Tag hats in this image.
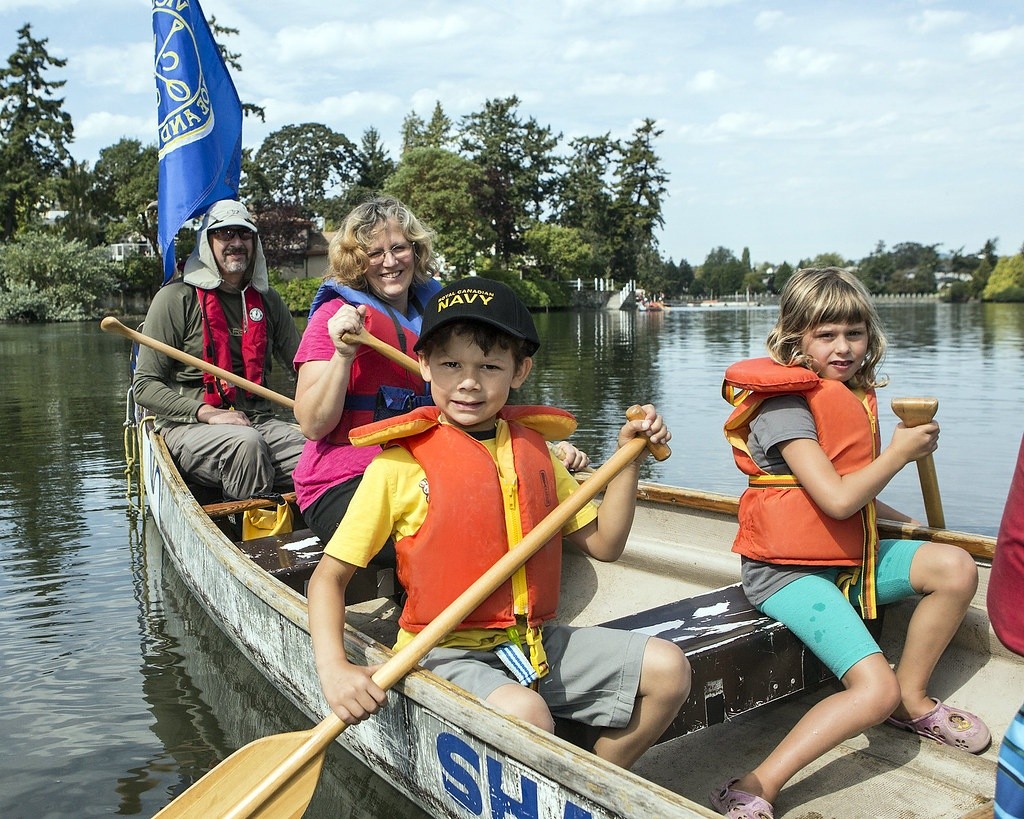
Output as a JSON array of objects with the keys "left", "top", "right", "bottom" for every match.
[
  {"left": 182, "top": 199, "right": 269, "bottom": 292},
  {"left": 412, "top": 276, "right": 540, "bottom": 358}
]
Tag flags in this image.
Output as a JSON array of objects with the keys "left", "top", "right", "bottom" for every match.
[{"left": 153, "top": 0, "right": 243, "bottom": 288}]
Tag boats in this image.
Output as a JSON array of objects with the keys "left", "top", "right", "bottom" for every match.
[{"left": 132, "top": 383, "right": 1024, "bottom": 819}]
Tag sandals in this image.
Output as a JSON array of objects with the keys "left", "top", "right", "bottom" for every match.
[
  {"left": 885, "top": 694, "right": 991, "bottom": 754},
  {"left": 708, "top": 775, "right": 774, "bottom": 819}
]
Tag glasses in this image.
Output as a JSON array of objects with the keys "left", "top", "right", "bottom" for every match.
[
  {"left": 209, "top": 227, "right": 253, "bottom": 241},
  {"left": 367, "top": 242, "right": 416, "bottom": 266}
]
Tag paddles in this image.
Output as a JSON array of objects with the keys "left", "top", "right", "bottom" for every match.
[
  {"left": 147, "top": 399, "right": 674, "bottom": 819},
  {"left": 337, "top": 321, "right": 600, "bottom": 477},
  {"left": 98, "top": 315, "right": 297, "bottom": 411},
  {"left": 886, "top": 394, "right": 953, "bottom": 530}
]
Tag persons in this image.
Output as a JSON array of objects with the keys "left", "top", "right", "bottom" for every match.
[
  {"left": 294, "top": 199, "right": 591, "bottom": 554},
  {"left": 306, "top": 274, "right": 692, "bottom": 772},
  {"left": 131, "top": 199, "right": 303, "bottom": 516},
  {"left": 708, "top": 265, "right": 992, "bottom": 813}
]
